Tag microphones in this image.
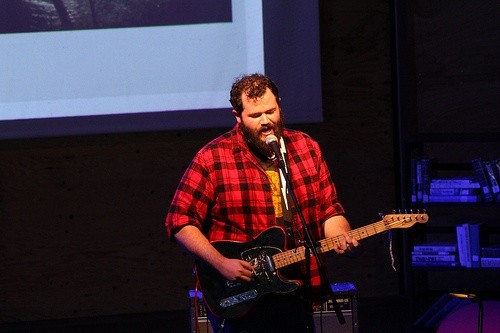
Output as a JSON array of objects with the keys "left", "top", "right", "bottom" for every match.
[{"left": 266, "top": 134, "right": 286, "bottom": 167}]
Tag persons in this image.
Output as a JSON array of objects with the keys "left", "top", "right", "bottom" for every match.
[{"left": 166, "top": 70, "right": 359, "bottom": 333}]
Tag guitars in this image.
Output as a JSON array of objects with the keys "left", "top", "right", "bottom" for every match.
[{"left": 193, "top": 208, "right": 431, "bottom": 321}]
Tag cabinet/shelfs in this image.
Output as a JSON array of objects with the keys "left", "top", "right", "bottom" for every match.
[{"left": 389, "top": 2, "right": 500, "bottom": 331}]
